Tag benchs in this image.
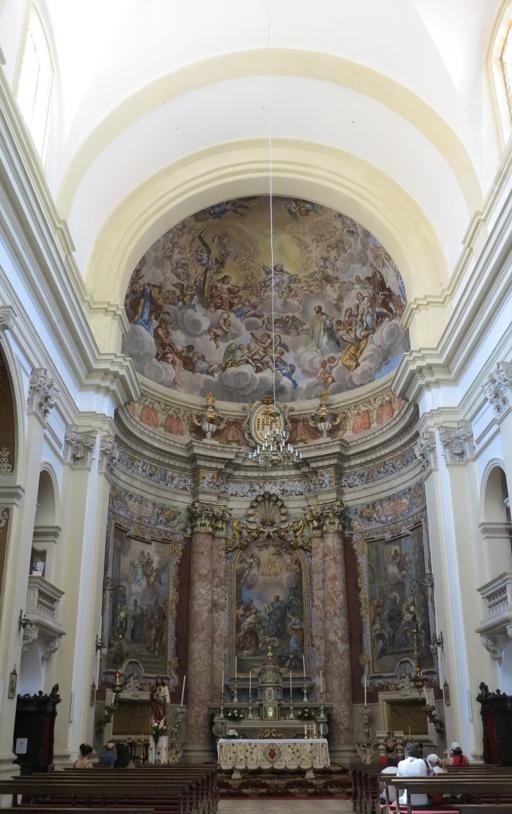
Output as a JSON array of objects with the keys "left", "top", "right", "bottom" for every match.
[
  {"left": 0, "top": 760, "right": 219, "bottom": 813},
  {"left": 351, "top": 761, "right": 512, "bottom": 814}
]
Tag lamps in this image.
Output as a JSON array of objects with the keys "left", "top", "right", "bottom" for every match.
[{"left": 247, "top": 429, "right": 301, "bottom": 470}]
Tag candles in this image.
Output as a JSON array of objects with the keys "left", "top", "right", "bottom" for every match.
[
  {"left": 249, "top": 670, "right": 252, "bottom": 692},
  {"left": 289, "top": 669, "right": 292, "bottom": 695},
  {"left": 319, "top": 670, "right": 323, "bottom": 693},
  {"left": 364, "top": 675, "right": 367, "bottom": 707},
  {"left": 302, "top": 654, "right": 306, "bottom": 682},
  {"left": 234, "top": 656, "right": 238, "bottom": 678},
  {"left": 180, "top": 675, "right": 187, "bottom": 709},
  {"left": 221, "top": 671, "right": 225, "bottom": 692}
]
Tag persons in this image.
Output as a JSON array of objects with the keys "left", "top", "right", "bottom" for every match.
[
  {"left": 114, "top": 745, "right": 135, "bottom": 769},
  {"left": 149, "top": 677, "right": 170, "bottom": 729},
  {"left": 378, "top": 756, "right": 404, "bottom": 805},
  {"left": 98, "top": 742, "right": 116, "bottom": 768},
  {"left": 74, "top": 743, "right": 94, "bottom": 769},
  {"left": 392, "top": 743, "right": 428, "bottom": 806},
  {"left": 444, "top": 743, "right": 469, "bottom": 768},
  {"left": 424, "top": 754, "right": 448, "bottom": 775}
]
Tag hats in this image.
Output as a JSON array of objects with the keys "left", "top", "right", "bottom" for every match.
[{"left": 450, "top": 742, "right": 461, "bottom": 751}]
